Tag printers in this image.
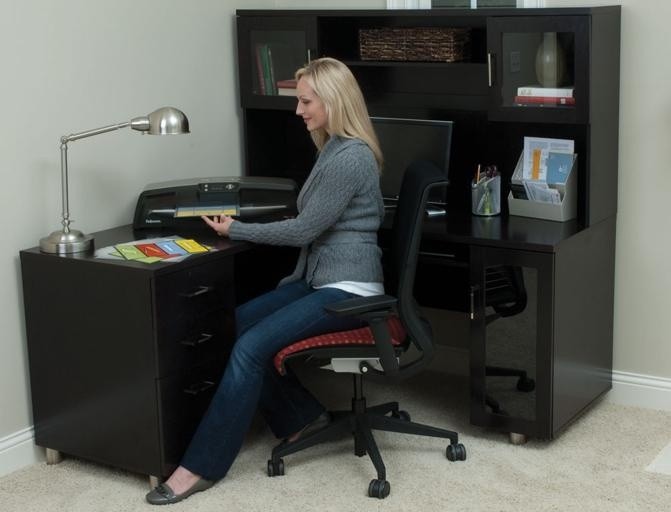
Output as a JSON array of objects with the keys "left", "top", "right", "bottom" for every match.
[{"left": 132, "top": 176, "right": 300, "bottom": 241}]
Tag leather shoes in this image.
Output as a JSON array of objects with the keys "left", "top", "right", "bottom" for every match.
[
  {"left": 272, "top": 412, "right": 328, "bottom": 456},
  {"left": 146, "top": 477, "right": 214, "bottom": 504}
]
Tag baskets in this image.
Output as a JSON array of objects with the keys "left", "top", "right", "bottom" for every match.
[{"left": 359, "top": 27, "right": 463, "bottom": 62}]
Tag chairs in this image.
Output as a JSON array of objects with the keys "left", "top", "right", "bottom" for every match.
[{"left": 265, "top": 158, "right": 467, "bottom": 501}]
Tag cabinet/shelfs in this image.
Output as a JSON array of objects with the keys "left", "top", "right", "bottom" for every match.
[
  {"left": 17, "top": 225, "right": 235, "bottom": 489},
  {"left": 237, "top": 6, "right": 622, "bottom": 225},
  {"left": 463, "top": 226, "right": 616, "bottom": 444}
]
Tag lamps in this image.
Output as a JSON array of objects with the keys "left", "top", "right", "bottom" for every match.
[{"left": 38, "top": 106, "right": 189, "bottom": 256}]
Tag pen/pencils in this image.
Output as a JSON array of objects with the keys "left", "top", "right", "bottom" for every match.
[{"left": 475, "top": 164, "right": 497, "bottom": 215}]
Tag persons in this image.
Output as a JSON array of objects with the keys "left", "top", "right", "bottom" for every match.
[{"left": 145, "top": 57, "right": 389, "bottom": 507}]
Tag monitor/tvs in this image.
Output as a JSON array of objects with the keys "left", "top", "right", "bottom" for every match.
[{"left": 368, "top": 116, "right": 453, "bottom": 217}]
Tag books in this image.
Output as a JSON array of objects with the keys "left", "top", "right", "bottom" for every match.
[
  {"left": 513, "top": 84, "right": 578, "bottom": 111},
  {"left": 250, "top": 39, "right": 303, "bottom": 98}
]
